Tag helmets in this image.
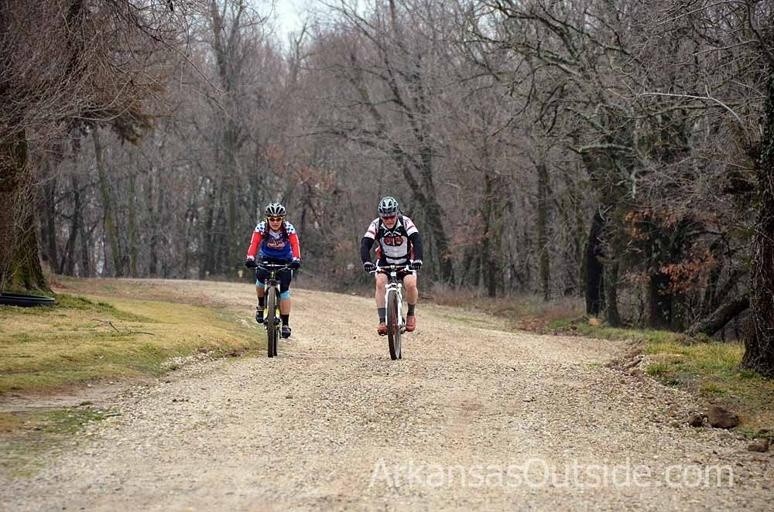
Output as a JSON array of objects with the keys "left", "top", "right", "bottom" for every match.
[
  {"left": 376, "top": 195, "right": 400, "bottom": 218},
  {"left": 263, "top": 202, "right": 287, "bottom": 217}
]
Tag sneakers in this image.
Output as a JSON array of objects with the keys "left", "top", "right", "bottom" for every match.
[
  {"left": 377, "top": 323, "right": 387, "bottom": 335},
  {"left": 406, "top": 312, "right": 416, "bottom": 333},
  {"left": 281, "top": 324, "right": 291, "bottom": 339},
  {"left": 255, "top": 307, "right": 265, "bottom": 323}
]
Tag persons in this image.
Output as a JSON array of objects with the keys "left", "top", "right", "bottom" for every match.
[
  {"left": 244, "top": 201, "right": 301, "bottom": 339},
  {"left": 360, "top": 196, "right": 424, "bottom": 334}
]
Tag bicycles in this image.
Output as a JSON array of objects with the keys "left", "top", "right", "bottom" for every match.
[
  {"left": 369, "top": 262, "right": 420, "bottom": 360},
  {"left": 255, "top": 261, "right": 295, "bottom": 358}
]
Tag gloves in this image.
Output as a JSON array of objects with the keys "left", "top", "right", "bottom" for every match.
[
  {"left": 364, "top": 261, "right": 378, "bottom": 272},
  {"left": 245, "top": 259, "right": 260, "bottom": 271},
  {"left": 409, "top": 258, "right": 422, "bottom": 271},
  {"left": 286, "top": 261, "right": 301, "bottom": 272}
]
